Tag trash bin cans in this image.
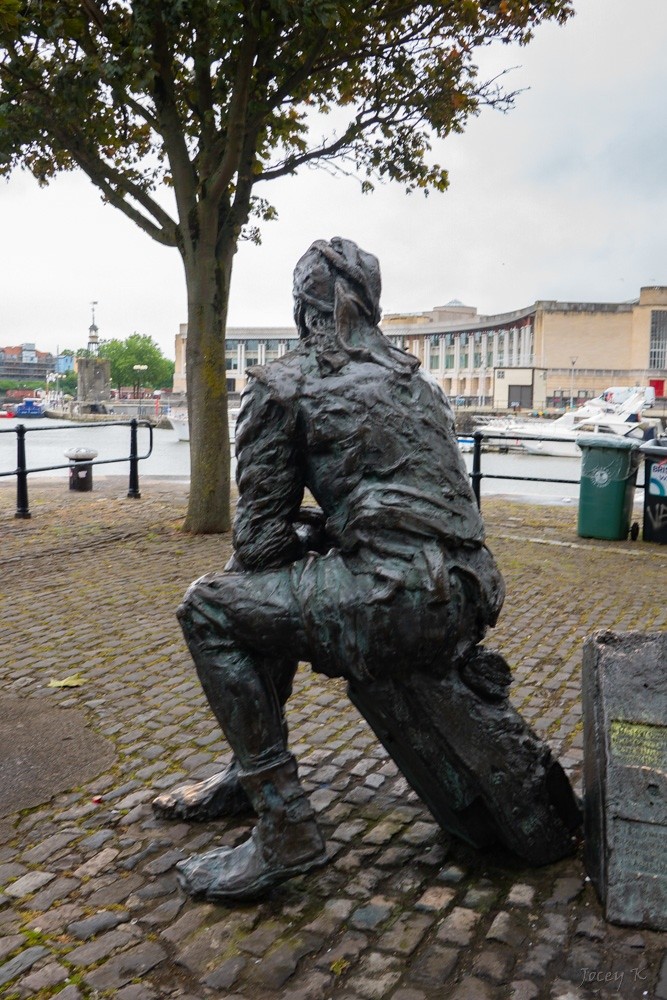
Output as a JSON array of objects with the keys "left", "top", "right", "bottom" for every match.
[
  {"left": 639, "top": 439, "right": 667, "bottom": 543},
  {"left": 576, "top": 437, "right": 641, "bottom": 540},
  {"left": 64, "top": 447, "right": 99, "bottom": 493}
]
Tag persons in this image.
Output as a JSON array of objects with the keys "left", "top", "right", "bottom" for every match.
[{"left": 153, "top": 235, "right": 506, "bottom": 906}]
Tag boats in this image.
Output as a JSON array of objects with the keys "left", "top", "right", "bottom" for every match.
[
  {"left": 17, "top": 398, "right": 46, "bottom": 418},
  {"left": 0, "top": 403, "right": 16, "bottom": 417},
  {"left": 168, "top": 408, "right": 238, "bottom": 444},
  {"left": 475, "top": 387, "right": 667, "bottom": 457},
  {"left": 459, "top": 435, "right": 475, "bottom": 451}
]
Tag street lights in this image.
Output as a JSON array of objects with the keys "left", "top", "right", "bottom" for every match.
[
  {"left": 46, "top": 372, "right": 67, "bottom": 404},
  {"left": 570, "top": 355, "right": 579, "bottom": 407},
  {"left": 133, "top": 365, "right": 148, "bottom": 398}
]
